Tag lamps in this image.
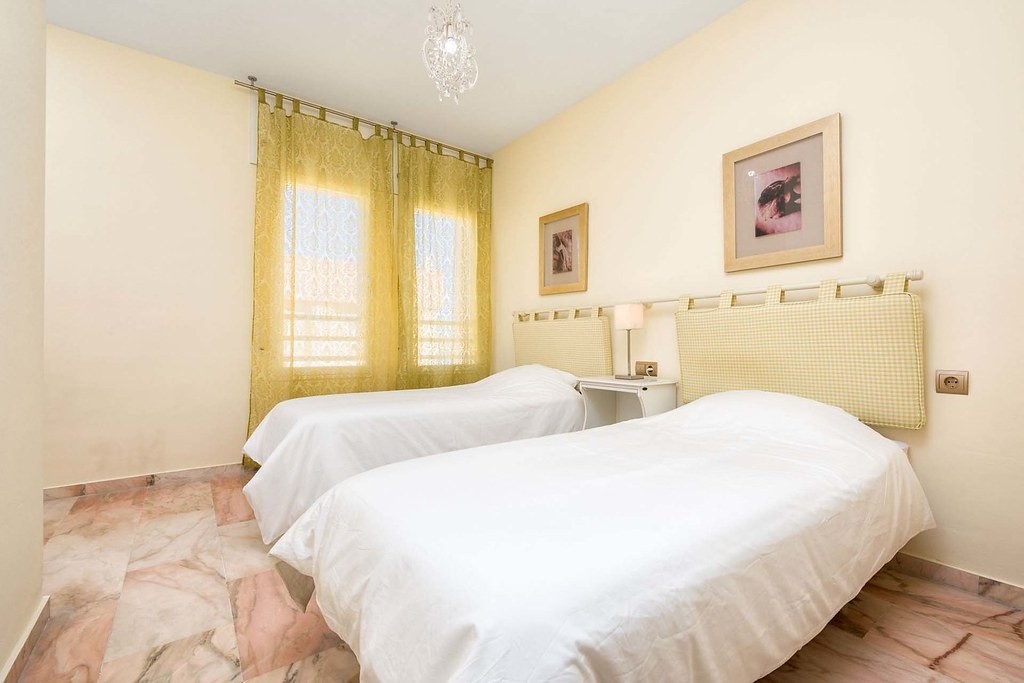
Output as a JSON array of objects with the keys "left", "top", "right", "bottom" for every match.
[
  {"left": 613, "top": 304, "right": 645, "bottom": 380},
  {"left": 421, "top": 0, "right": 479, "bottom": 107}
]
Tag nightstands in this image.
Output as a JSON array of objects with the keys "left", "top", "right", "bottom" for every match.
[{"left": 577, "top": 375, "right": 679, "bottom": 431}]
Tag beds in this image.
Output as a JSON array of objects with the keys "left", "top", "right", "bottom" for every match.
[
  {"left": 239, "top": 306, "right": 614, "bottom": 549},
  {"left": 270, "top": 269, "right": 938, "bottom": 683}
]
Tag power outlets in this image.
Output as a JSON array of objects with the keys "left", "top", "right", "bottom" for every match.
[
  {"left": 635, "top": 361, "right": 657, "bottom": 377},
  {"left": 935, "top": 370, "right": 968, "bottom": 395}
]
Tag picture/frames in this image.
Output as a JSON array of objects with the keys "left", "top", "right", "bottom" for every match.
[
  {"left": 722, "top": 113, "right": 843, "bottom": 273},
  {"left": 539, "top": 203, "right": 589, "bottom": 295}
]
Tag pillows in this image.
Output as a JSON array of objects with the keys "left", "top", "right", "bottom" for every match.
[{"left": 536, "top": 363, "right": 580, "bottom": 388}]
